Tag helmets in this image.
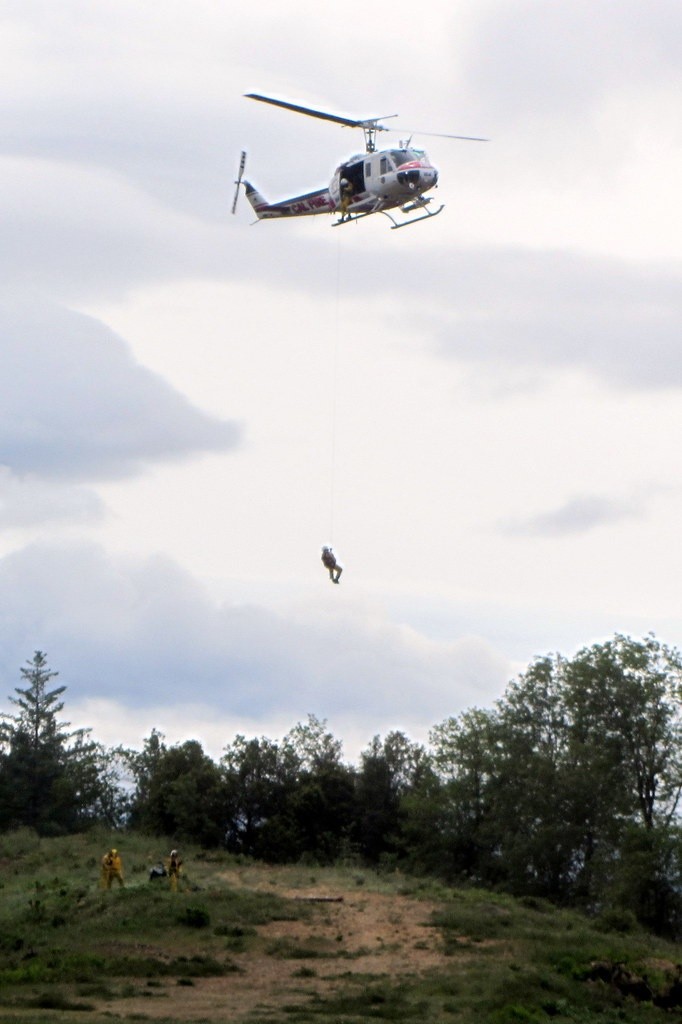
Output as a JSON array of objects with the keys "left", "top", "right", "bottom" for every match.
[
  {"left": 322, "top": 546, "right": 329, "bottom": 551},
  {"left": 112, "top": 849, "right": 117, "bottom": 854},
  {"left": 170, "top": 850, "right": 177, "bottom": 856}
]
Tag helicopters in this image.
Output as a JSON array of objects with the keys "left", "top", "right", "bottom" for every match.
[{"left": 228, "top": 93, "right": 491, "bottom": 230}]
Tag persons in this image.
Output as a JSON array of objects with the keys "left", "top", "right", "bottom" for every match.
[
  {"left": 322, "top": 546, "right": 343, "bottom": 584},
  {"left": 151, "top": 861, "right": 166, "bottom": 879},
  {"left": 337, "top": 178, "right": 353, "bottom": 223},
  {"left": 105, "top": 849, "right": 124, "bottom": 887},
  {"left": 170, "top": 849, "right": 182, "bottom": 891}
]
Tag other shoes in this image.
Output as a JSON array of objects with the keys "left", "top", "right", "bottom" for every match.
[{"left": 332, "top": 579, "right": 339, "bottom": 583}]
